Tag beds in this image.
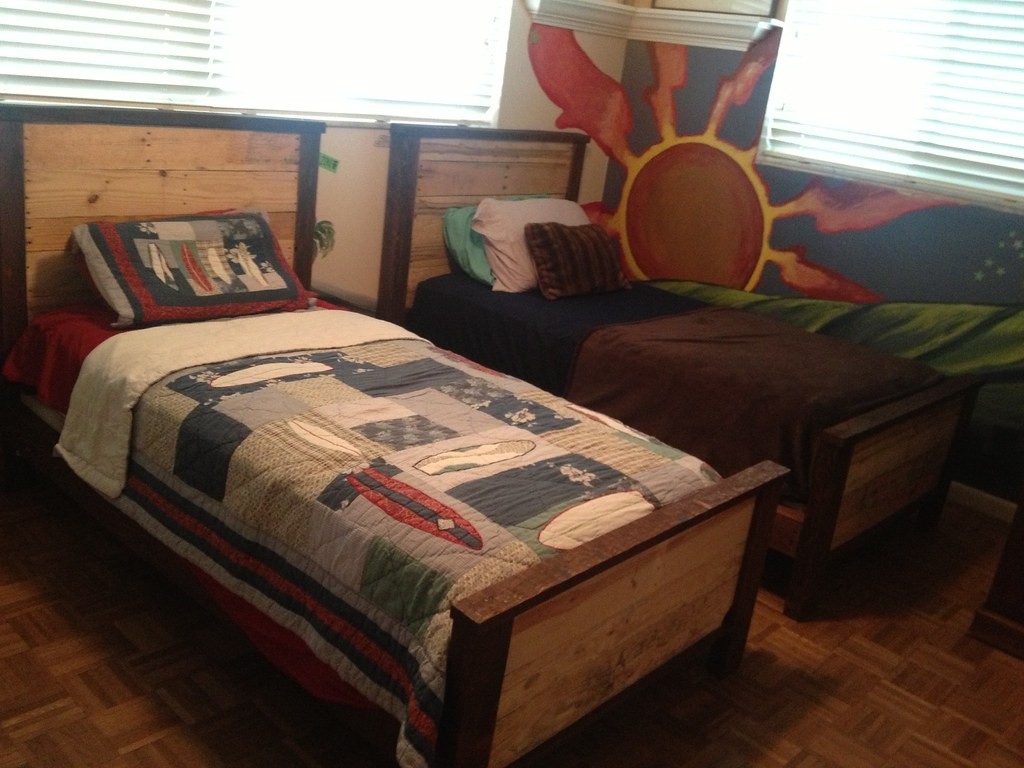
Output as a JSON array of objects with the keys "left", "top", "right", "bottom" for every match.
[
  {"left": 375, "top": 124, "right": 981, "bottom": 620},
  {"left": 0, "top": 104, "right": 793, "bottom": 768}
]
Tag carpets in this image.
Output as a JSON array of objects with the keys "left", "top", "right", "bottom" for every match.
[{"left": 967, "top": 502, "right": 1024, "bottom": 661}]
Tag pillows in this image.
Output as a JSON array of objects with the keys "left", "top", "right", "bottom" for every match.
[
  {"left": 62, "top": 208, "right": 311, "bottom": 328},
  {"left": 440, "top": 195, "right": 550, "bottom": 285},
  {"left": 472, "top": 200, "right": 591, "bottom": 292},
  {"left": 524, "top": 222, "right": 634, "bottom": 301}
]
